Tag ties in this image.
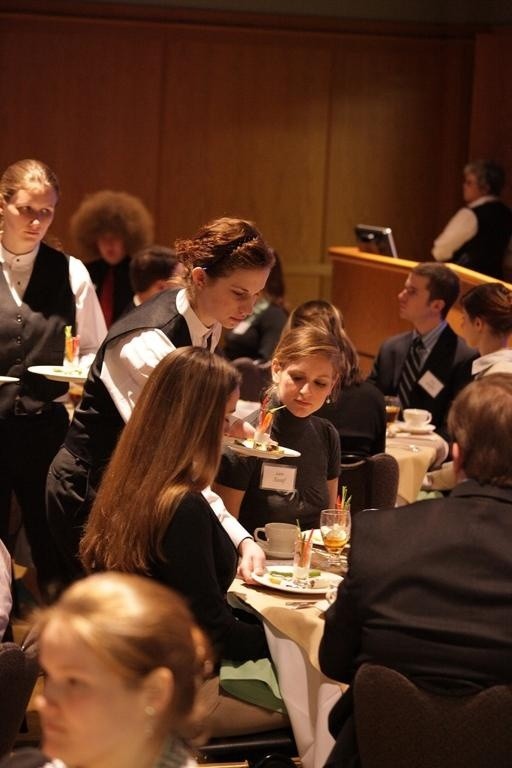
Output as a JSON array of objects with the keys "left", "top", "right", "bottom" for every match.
[{"left": 396, "top": 336, "right": 425, "bottom": 421}]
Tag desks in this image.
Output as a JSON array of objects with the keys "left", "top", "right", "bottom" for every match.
[{"left": 333, "top": 245, "right": 511, "bottom": 381}]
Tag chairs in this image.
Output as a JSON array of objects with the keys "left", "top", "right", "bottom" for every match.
[
  {"left": 352, "top": 662, "right": 512, "bottom": 768},
  {"left": 335, "top": 455, "right": 402, "bottom": 514},
  {"left": 193, "top": 734, "right": 300, "bottom": 766},
  {"left": 0, "top": 639, "right": 40, "bottom": 767},
  {"left": 227, "top": 358, "right": 278, "bottom": 402}
]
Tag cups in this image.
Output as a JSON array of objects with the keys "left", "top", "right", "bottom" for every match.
[
  {"left": 335, "top": 502, "right": 350, "bottom": 526},
  {"left": 403, "top": 409, "right": 432, "bottom": 427},
  {"left": 320, "top": 509, "right": 352, "bottom": 569},
  {"left": 383, "top": 396, "right": 401, "bottom": 439},
  {"left": 293, "top": 539, "right": 313, "bottom": 569},
  {"left": 254, "top": 523, "right": 300, "bottom": 553}
]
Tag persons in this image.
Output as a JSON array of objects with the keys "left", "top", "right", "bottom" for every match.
[
  {"left": 432, "top": 159, "right": 512, "bottom": 279},
  {"left": 211, "top": 329, "right": 342, "bottom": 546},
  {"left": 35, "top": 571, "right": 206, "bottom": 768},
  {"left": 45, "top": 218, "right": 279, "bottom": 584},
  {"left": 79, "top": 347, "right": 290, "bottom": 739},
  {"left": 121, "top": 246, "right": 183, "bottom": 318},
  {"left": 0, "top": 161, "right": 110, "bottom": 602},
  {"left": 321, "top": 375, "right": 512, "bottom": 768},
  {"left": 71, "top": 191, "right": 152, "bottom": 326},
  {"left": 280, "top": 298, "right": 385, "bottom": 458},
  {"left": 363, "top": 262, "right": 481, "bottom": 464},
  {"left": 223, "top": 246, "right": 288, "bottom": 363},
  {"left": 418, "top": 283, "right": 512, "bottom": 491}
]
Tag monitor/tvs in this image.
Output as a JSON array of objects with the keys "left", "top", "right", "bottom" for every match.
[{"left": 355, "top": 223, "right": 398, "bottom": 259}]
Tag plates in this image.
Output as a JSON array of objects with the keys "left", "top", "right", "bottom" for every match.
[
  {"left": 28, "top": 366, "right": 88, "bottom": 383},
  {"left": 251, "top": 565, "right": 344, "bottom": 593},
  {"left": 398, "top": 424, "right": 435, "bottom": 433},
  {"left": 264, "top": 551, "right": 294, "bottom": 558},
  {"left": 224, "top": 438, "right": 301, "bottom": 459},
  {"left": 301, "top": 529, "right": 351, "bottom": 548}
]
